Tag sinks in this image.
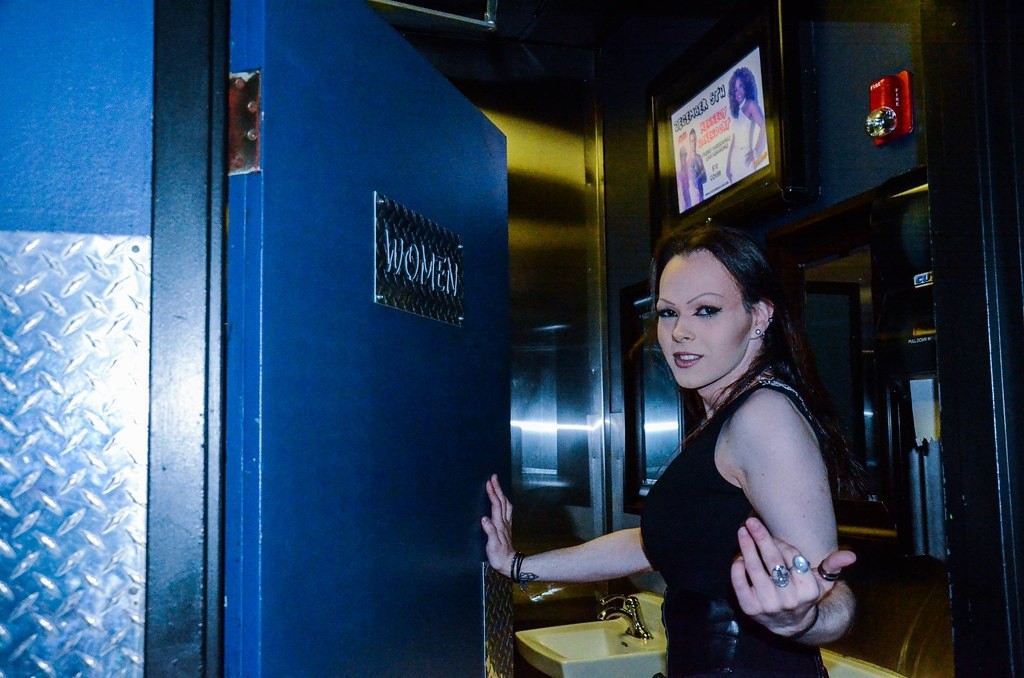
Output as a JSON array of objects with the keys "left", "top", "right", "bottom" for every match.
[{"left": 515, "top": 624, "right": 666, "bottom": 678}]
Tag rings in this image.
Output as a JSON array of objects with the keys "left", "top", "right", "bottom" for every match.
[
  {"left": 770, "top": 564, "right": 791, "bottom": 587},
  {"left": 818, "top": 559, "right": 841, "bottom": 581},
  {"left": 789, "top": 555, "right": 810, "bottom": 573}
]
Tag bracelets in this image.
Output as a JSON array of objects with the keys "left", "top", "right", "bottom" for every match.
[
  {"left": 510, "top": 551, "right": 528, "bottom": 589},
  {"left": 789, "top": 606, "right": 821, "bottom": 640}
]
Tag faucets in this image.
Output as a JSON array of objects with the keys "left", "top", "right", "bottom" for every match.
[{"left": 598, "top": 594, "right": 654, "bottom": 640}]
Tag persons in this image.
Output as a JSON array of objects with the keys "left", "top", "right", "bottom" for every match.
[
  {"left": 482, "top": 226, "right": 856, "bottom": 678},
  {"left": 725, "top": 68, "right": 764, "bottom": 184},
  {"left": 676, "top": 129, "right": 709, "bottom": 212}
]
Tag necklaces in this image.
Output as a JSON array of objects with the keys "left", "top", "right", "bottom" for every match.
[{"left": 701, "top": 367, "right": 770, "bottom": 425}]
